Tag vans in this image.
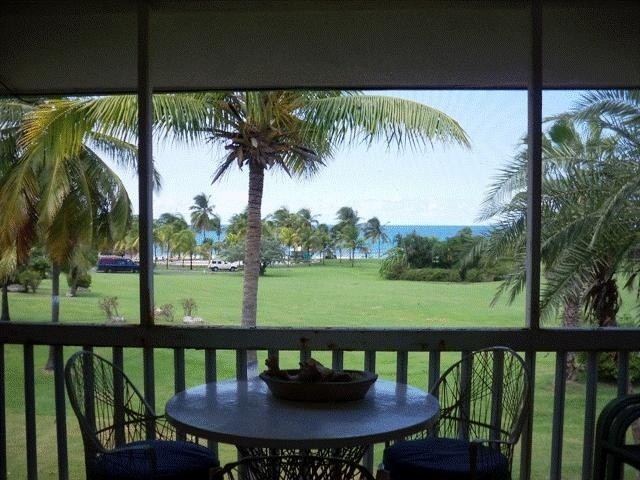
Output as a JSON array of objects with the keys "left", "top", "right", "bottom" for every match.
[{"left": 96, "top": 257, "right": 140, "bottom": 273}]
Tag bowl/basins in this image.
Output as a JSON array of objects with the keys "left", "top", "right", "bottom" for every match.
[{"left": 258, "top": 368, "right": 378, "bottom": 402}]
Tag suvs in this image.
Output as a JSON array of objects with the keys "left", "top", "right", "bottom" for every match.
[{"left": 208, "top": 259, "right": 236, "bottom": 272}]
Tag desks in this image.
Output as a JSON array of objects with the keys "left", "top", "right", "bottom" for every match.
[{"left": 164, "top": 375, "right": 441, "bottom": 464}]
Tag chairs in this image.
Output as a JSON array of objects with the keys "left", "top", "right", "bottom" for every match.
[
  {"left": 375, "top": 346, "right": 533, "bottom": 479},
  {"left": 215, "top": 454, "right": 374, "bottom": 479},
  {"left": 64, "top": 351, "right": 221, "bottom": 479}
]
